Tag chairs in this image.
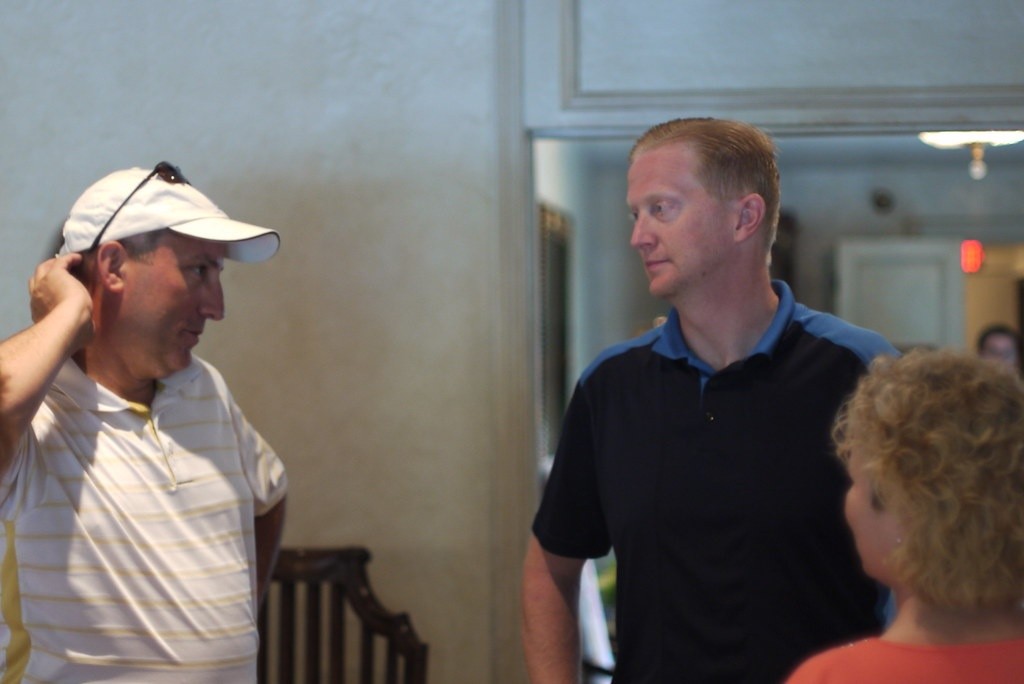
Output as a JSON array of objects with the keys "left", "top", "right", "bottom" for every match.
[{"left": 255, "top": 546, "right": 429, "bottom": 684}]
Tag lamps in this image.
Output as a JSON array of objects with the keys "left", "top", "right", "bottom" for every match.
[{"left": 969, "top": 142, "right": 987, "bottom": 181}]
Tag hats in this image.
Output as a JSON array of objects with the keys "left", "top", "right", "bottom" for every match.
[{"left": 55, "top": 166, "right": 281, "bottom": 265}]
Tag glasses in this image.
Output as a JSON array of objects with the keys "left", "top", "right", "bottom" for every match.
[{"left": 87, "top": 162, "right": 189, "bottom": 253}]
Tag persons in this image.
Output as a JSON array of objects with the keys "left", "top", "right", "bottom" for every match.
[
  {"left": 976, "top": 322, "right": 1016, "bottom": 365},
  {"left": 784, "top": 347, "right": 1024, "bottom": 684},
  {"left": 0, "top": 158, "right": 289, "bottom": 684},
  {"left": 522, "top": 116, "right": 898, "bottom": 684}
]
